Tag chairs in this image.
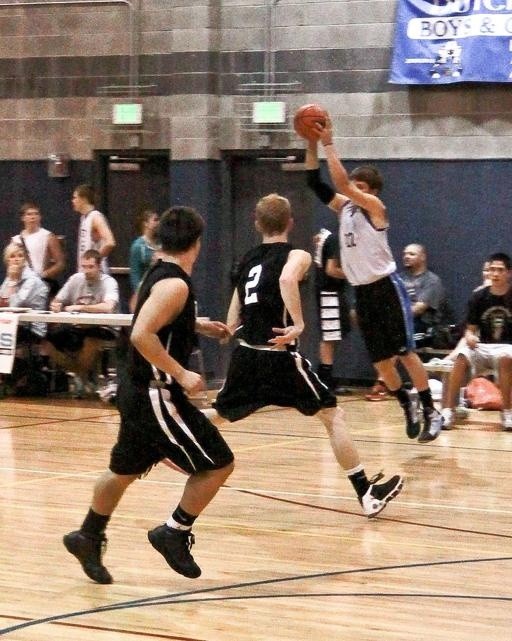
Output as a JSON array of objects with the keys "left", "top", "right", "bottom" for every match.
[
  {"left": 40, "top": 276, "right": 60, "bottom": 308},
  {"left": 189, "top": 300, "right": 207, "bottom": 395}
]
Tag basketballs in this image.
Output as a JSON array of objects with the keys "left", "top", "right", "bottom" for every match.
[{"left": 293, "top": 104, "right": 331, "bottom": 142}]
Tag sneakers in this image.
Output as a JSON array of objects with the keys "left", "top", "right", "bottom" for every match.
[
  {"left": 61, "top": 526, "right": 116, "bottom": 585},
  {"left": 415, "top": 411, "right": 445, "bottom": 447},
  {"left": 401, "top": 398, "right": 422, "bottom": 444},
  {"left": 363, "top": 382, "right": 392, "bottom": 400},
  {"left": 354, "top": 467, "right": 409, "bottom": 524},
  {"left": 496, "top": 406, "right": 512, "bottom": 433},
  {"left": 437, "top": 405, "right": 456, "bottom": 431},
  {"left": 145, "top": 521, "right": 203, "bottom": 580}
]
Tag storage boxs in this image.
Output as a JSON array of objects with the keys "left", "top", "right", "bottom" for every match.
[{"left": 101, "top": 282, "right": 129, "bottom": 377}]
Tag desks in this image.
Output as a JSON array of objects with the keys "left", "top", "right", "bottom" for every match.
[{"left": 0, "top": 310, "right": 133, "bottom": 328}]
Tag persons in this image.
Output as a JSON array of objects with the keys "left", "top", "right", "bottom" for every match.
[
  {"left": 200, "top": 195, "right": 404, "bottom": 519},
  {"left": 365, "top": 243, "right": 444, "bottom": 401},
  {"left": 1, "top": 242, "right": 51, "bottom": 398},
  {"left": 128, "top": 209, "right": 163, "bottom": 293},
  {"left": 7, "top": 202, "right": 66, "bottom": 280},
  {"left": 313, "top": 228, "right": 356, "bottom": 396},
  {"left": 438, "top": 254, "right": 512, "bottom": 430},
  {"left": 50, "top": 249, "right": 121, "bottom": 398},
  {"left": 64, "top": 207, "right": 235, "bottom": 584},
  {"left": 70, "top": 182, "right": 115, "bottom": 274},
  {"left": 306, "top": 114, "right": 447, "bottom": 443}
]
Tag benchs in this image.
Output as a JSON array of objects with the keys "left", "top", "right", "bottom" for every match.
[
  {"left": 415, "top": 348, "right": 456, "bottom": 356},
  {"left": 422, "top": 359, "right": 500, "bottom": 410}
]
